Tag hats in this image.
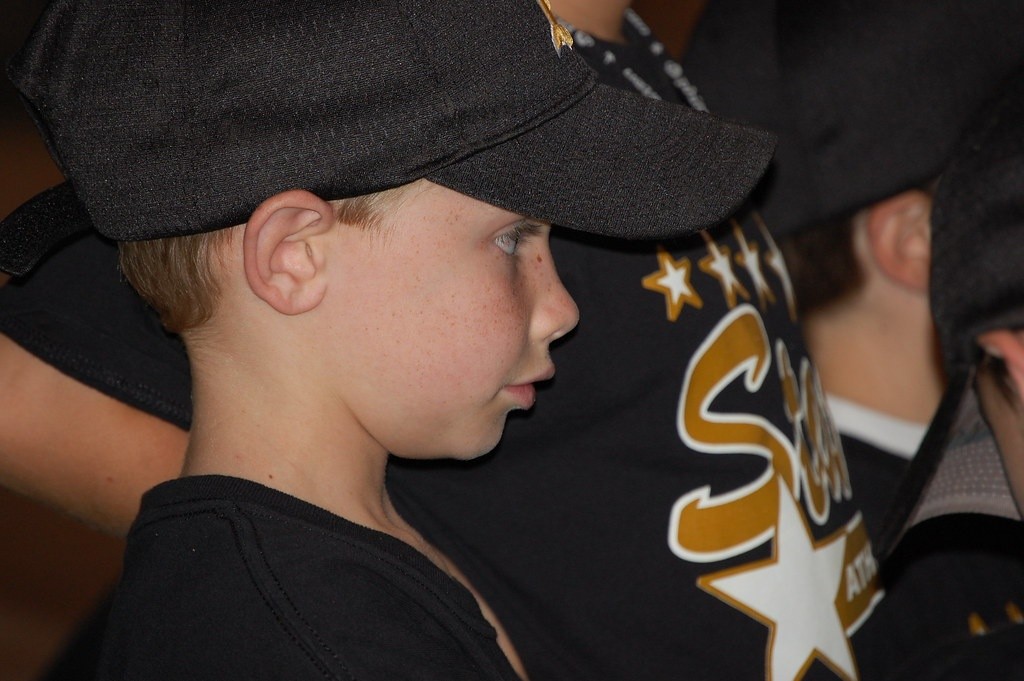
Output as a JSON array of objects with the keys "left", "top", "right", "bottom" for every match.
[
  {"left": 7, "top": 1, "right": 780, "bottom": 239},
  {"left": 931, "top": 88, "right": 1024, "bottom": 388},
  {"left": 678, "top": 0, "right": 1024, "bottom": 234}
]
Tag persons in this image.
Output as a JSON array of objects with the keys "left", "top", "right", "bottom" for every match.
[{"left": 0, "top": 0, "right": 1023, "bottom": 681}]
